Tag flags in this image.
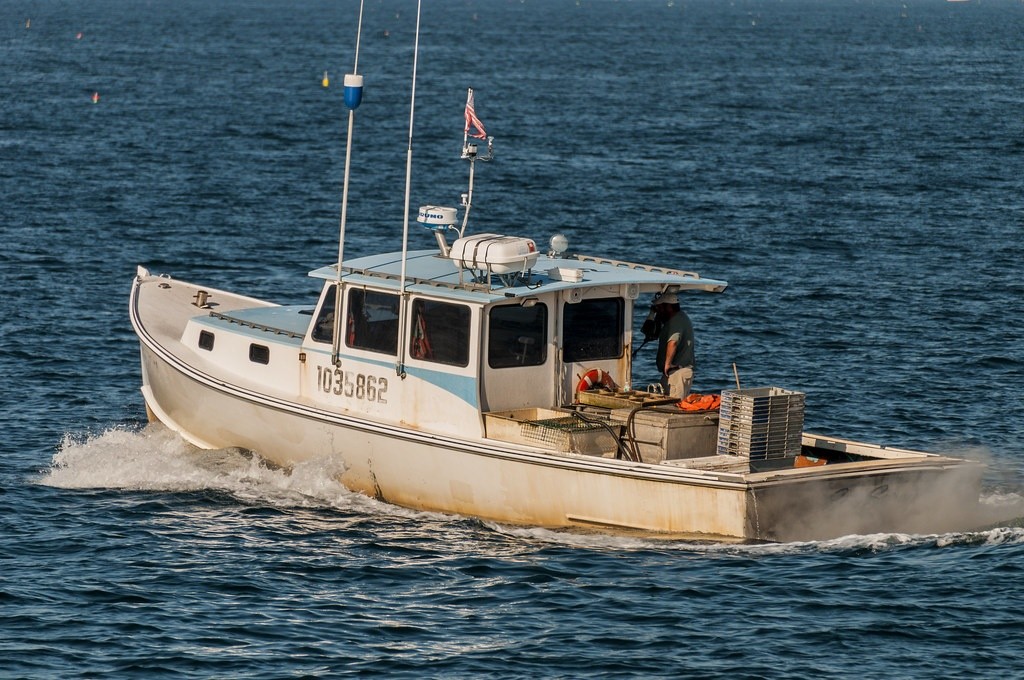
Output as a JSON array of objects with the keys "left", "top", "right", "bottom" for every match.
[{"left": 465, "top": 94, "right": 486, "bottom": 140}]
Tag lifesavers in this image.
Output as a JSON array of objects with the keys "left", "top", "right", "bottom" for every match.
[{"left": 576, "top": 369, "right": 614, "bottom": 397}]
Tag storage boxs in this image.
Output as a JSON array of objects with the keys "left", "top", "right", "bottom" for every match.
[{"left": 483, "top": 386, "right": 805, "bottom": 469}]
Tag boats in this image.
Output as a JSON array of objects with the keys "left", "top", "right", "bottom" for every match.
[{"left": 123, "top": 0, "right": 992, "bottom": 553}]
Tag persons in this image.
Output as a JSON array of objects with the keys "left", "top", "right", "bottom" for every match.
[{"left": 656, "top": 295, "right": 695, "bottom": 401}]
